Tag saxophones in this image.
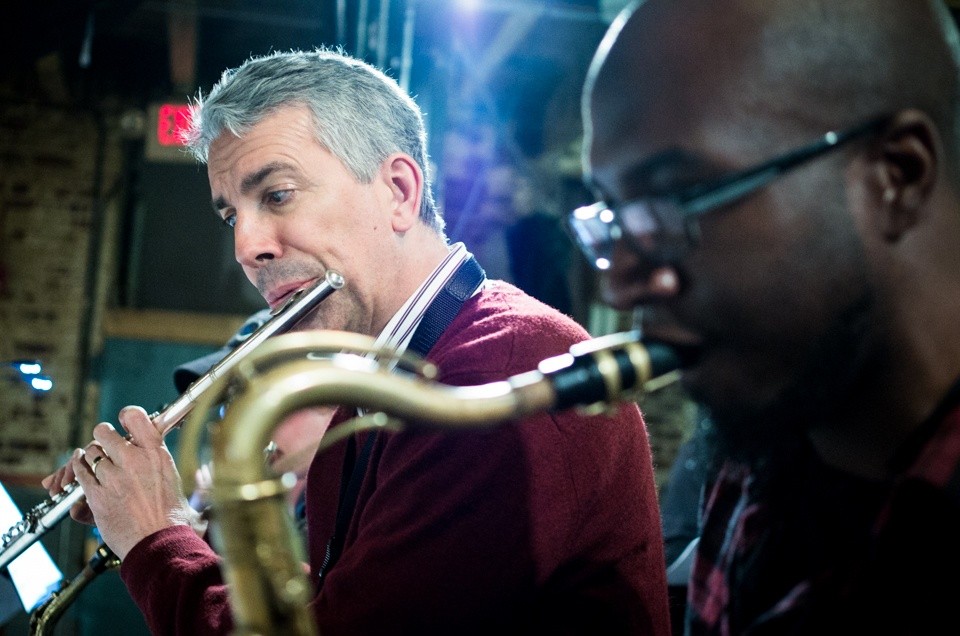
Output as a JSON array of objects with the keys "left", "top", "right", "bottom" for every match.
[{"left": 180, "top": 326, "right": 686, "bottom": 636}]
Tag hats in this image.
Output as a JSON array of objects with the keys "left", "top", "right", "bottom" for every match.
[{"left": 174, "top": 308, "right": 276, "bottom": 395}]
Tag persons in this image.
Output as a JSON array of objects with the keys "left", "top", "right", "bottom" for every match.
[{"left": 42, "top": 0, "right": 960, "bottom": 636}]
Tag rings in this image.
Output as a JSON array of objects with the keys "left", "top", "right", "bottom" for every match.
[{"left": 90, "top": 455, "right": 108, "bottom": 475}]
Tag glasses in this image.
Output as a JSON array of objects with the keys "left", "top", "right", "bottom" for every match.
[{"left": 559, "top": 102, "right": 945, "bottom": 275}]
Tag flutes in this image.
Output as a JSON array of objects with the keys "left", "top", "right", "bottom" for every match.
[{"left": 0, "top": 268, "right": 347, "bottom": 572}]
